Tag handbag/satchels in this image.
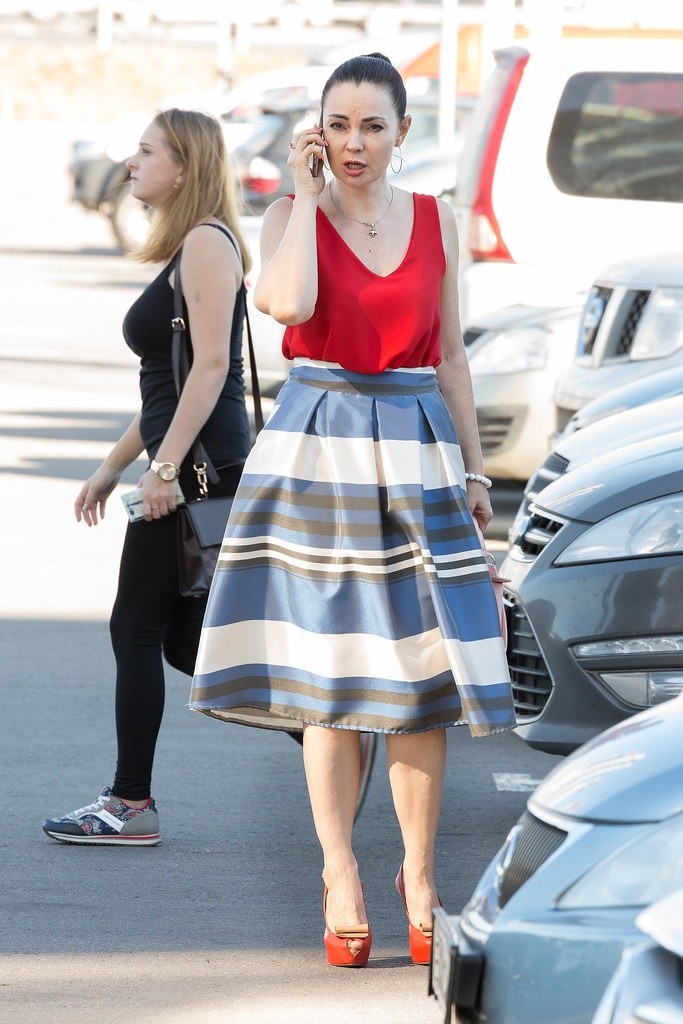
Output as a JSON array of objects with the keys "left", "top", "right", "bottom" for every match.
[
  {"left": 175, "top": 495, "right": 235, "bottom": 598},
  {"left": 471, "top": 512, "right": 508, "bottom": 653}
]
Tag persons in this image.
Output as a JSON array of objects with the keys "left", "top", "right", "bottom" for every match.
[
  {"left": 44, "top": 109, "right": 379, "bottom": 846},
  {"left": 188, "top": 52, "right": 518, "bottom": 969}
]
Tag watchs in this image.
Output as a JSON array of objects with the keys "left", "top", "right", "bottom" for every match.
[{"left": 151, "top": 460, "right": 180, "bottom": 481}]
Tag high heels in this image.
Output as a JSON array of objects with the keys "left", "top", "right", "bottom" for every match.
[
  {"left": 323, "top": 880, "right": 372, "bottom": 967},
  {"left": 395, "top": 864, "right": 444, "bottom": 965}
]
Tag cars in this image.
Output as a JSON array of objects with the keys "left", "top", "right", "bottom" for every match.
[
  {"left": 429, "top": 685, "right": 683, "bottom": 1024},
  {"left": 459, "top": 36, "right": 683, "bottom": 493},
  {"left": 99, "top": 77, "right": 492, "bottom": 263},
  {"left": 490, "top": 250, "right": 683, "bottom": 758}
]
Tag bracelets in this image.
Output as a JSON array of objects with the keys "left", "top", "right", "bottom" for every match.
[{"left": 465, "top": 472, "right": 492, "bottom": 488}]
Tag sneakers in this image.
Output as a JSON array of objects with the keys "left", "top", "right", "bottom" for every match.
[
  {"left": 42, "top": 786, "right": 162, "bottom": 846},
  {"left": 353, "top": 732, "right": 376, "bottom": 826}
]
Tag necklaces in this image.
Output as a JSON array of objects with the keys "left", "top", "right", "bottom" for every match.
[{"left": 328, "top": 179, "right": 394, "bottom": 237}]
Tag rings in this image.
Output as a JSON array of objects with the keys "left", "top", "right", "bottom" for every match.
[{"left": 289, "top": 143, "right": 295, "bottom": 149}]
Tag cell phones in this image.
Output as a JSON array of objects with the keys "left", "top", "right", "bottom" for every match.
[
  {"left": 122, "top": 478, "right": 185, "bottom": 523},
  {"left": 312, "top": 113, "right": 325, "bottom": 178}
]
